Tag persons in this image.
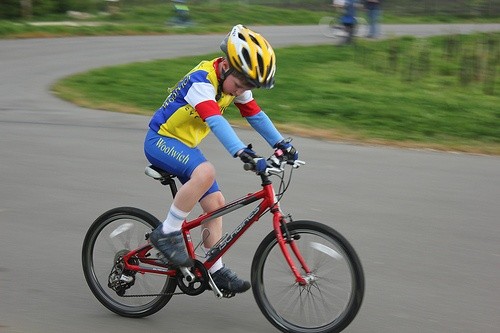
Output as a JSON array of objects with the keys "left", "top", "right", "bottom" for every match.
[
  {"left": 333, "top": 0, "right": 380, "bottom": 47},
  {"left": 144, "top": 22, "right": 301, "bottom": 294},
  {"left": 172, "top": 0, "right": 190, "bottom": 21}
]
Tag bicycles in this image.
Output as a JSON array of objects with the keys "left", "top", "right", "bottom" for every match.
[
  {"left": 82, "top": 138, "right": 365, "bottom": 333},
  {"left": 320, "top": 8, "right": 371, "bottom": 39}
]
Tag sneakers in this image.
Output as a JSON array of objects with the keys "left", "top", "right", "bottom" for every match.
[
  {"left": 147, "top": 223, "right": 194, "bottom": 267},
  {"left": 206, "top": 263, "right": 251, "bottom": 294}
]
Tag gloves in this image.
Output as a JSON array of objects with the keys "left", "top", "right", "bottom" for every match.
[
  {"left": 239, "top": 143, "right": 267, "bottom": 174},
  {"left": 277, "top": 140, "right": 298, "bottom": 161}
]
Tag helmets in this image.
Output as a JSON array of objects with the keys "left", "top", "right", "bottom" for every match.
[{"left": 220, "top": 24, "right": 276, "bottom": 89}]
